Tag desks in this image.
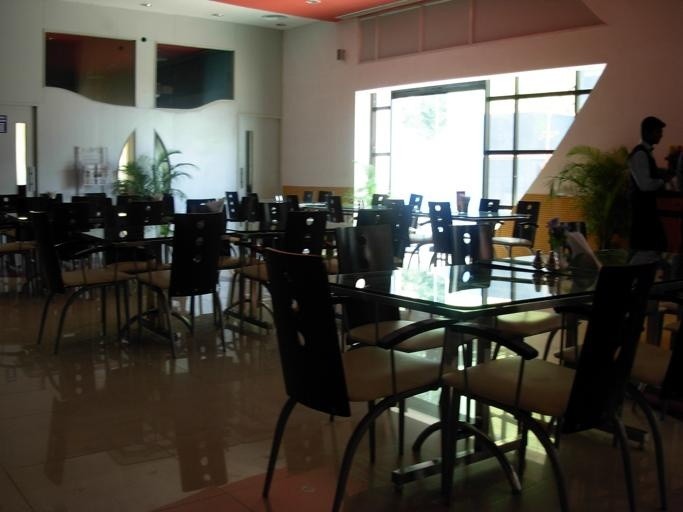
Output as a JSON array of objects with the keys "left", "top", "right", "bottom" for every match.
[{"left": 327, "top": 249, "right": 683, "bottom": 492}]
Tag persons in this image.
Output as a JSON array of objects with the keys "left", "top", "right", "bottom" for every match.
[{"left": 626, "top": 116, "right": 676, "bottom": 251}]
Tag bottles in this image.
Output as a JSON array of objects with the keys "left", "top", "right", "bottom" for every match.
[
  {"left": 531, "top": 249, "right": 543, "bottom": 272},
  {"left": 543, "top": 247, "right": 561, "bottom": 272}
]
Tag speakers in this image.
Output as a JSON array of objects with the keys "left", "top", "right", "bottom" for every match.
[{"left": 337, "top": 49, "right": 345, "bottom": 61}]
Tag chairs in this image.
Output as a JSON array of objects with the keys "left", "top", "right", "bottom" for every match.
[
  {"left": 448, "top": 261, "right": 668, "bottom": 512},
  {"left": 449, "top": 223, "right": 566, "bottom": 445},
  {"left": 331, "top": 222, "right": 471, "bottom": 457},
  {"left": 261, "top": 245, "right": 443, "bottom": 512},
  {"left": 270, "top": 187, "right": 541, "bottom": 254}
]
